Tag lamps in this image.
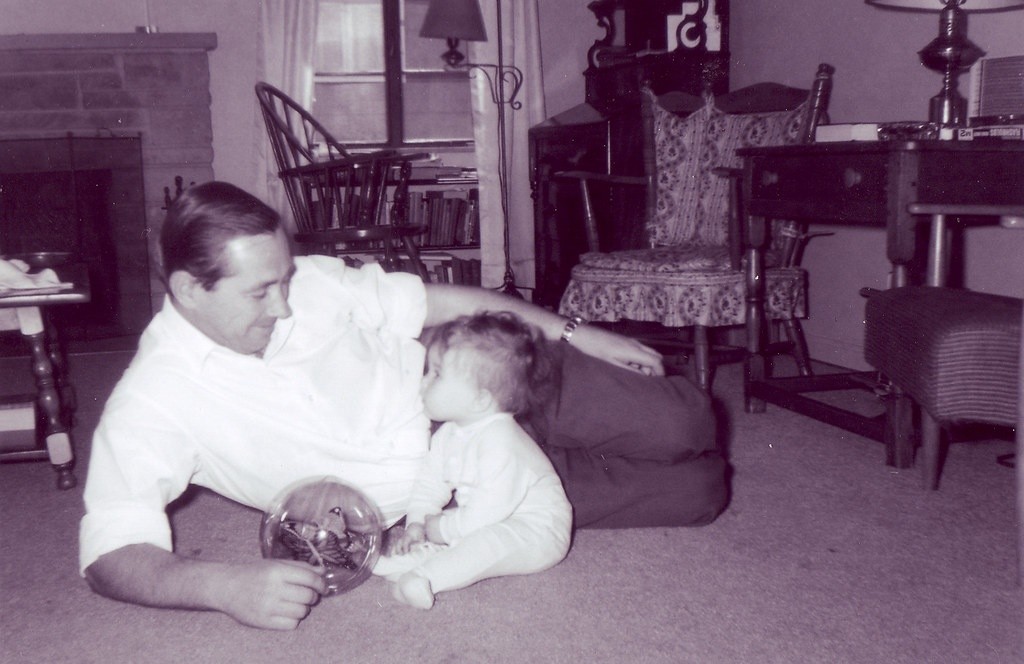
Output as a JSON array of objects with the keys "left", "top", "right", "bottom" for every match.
[
  {"left": 861, "top": 0, "right": 1024, "bottom": 128},
  {"left": 418, "top": 0, "right": 525, "bottom": 300}
]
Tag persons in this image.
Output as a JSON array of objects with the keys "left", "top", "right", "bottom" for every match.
[
  {"left": 372, "top": 313, "right": 575, "bottom": 609},
  {"left": 77, "top": 181, "right": 732, "bottom": 632}
]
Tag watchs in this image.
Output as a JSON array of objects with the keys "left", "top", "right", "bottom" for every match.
[{"left": 560, "top": 314, "right": 588, "bottom": 343}]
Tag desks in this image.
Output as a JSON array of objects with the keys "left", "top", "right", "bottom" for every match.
[
  {"left": 732, "top": 135, "right": 1024, "bottom": 469},
  {"left": 0, "top": 249, "right": 91, "bottom": 491}
]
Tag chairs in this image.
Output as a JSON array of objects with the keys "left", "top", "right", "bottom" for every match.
[
  {"left": 865, "top": 202, "right": 1024, "bottom": 493},
  {"left": 254, "top": 80, "right": 441, "bottom": 283},
  {"left": 551, "top": 57, "right": 836, "bottom": 400}
]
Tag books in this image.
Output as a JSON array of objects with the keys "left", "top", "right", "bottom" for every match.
[
  {"left": 308, "top": 152, "right": 481, "bottom": 291},
  {"left": 815, "top": 121, "right": 955, "bottom": 142},
  {"left": 958, "top": 124, "right": 1024, "bottom": 141}
]
